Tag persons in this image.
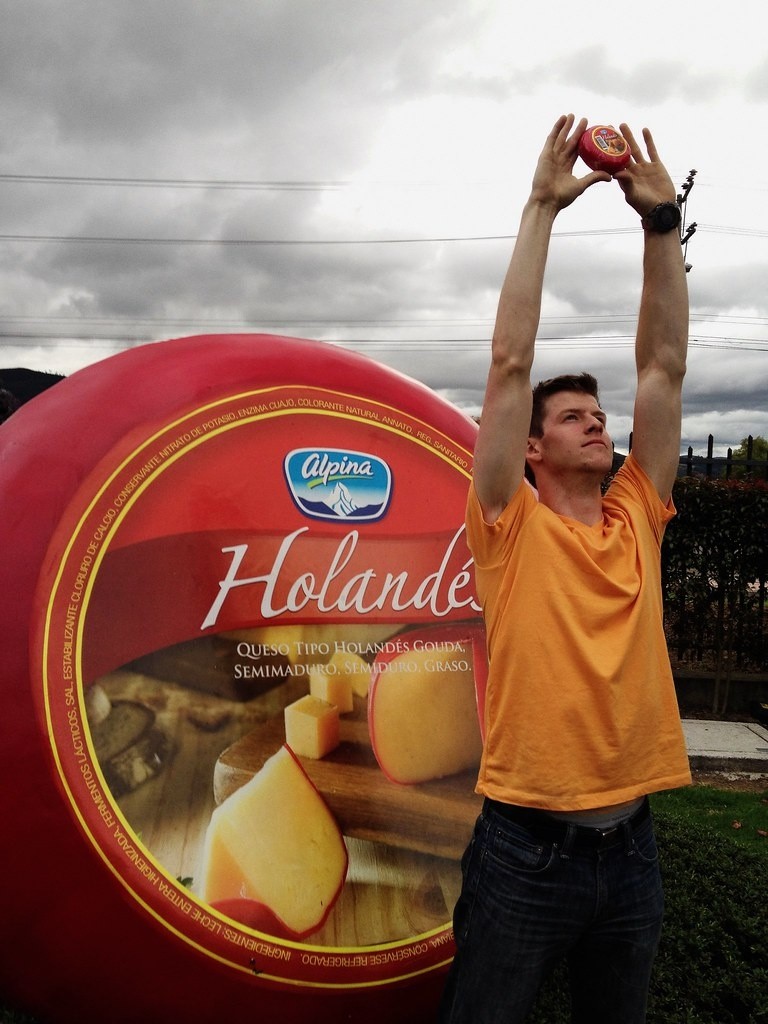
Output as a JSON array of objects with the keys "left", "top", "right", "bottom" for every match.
[{"left": 442, "top": 113, "right": 690, "bottom": 1024}]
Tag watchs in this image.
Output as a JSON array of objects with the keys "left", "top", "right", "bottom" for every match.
[{"left": 640, "top": 203, "right": 682, "bottom": 235}]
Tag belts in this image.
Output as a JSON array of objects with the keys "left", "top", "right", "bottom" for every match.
[{"left": 490, "top": 795, "right": 651, "bottom": 854}]
